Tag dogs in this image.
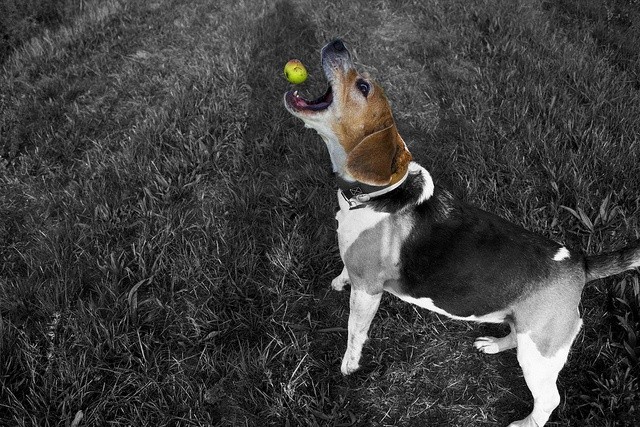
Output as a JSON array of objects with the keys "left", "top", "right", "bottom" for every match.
[{"left": 281, "top": 38, "right": 640, "bottom": 427}]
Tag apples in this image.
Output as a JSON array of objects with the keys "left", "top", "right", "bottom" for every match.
[{"left": 284, "top": 59, "right": 308, "bottom": 86}]
